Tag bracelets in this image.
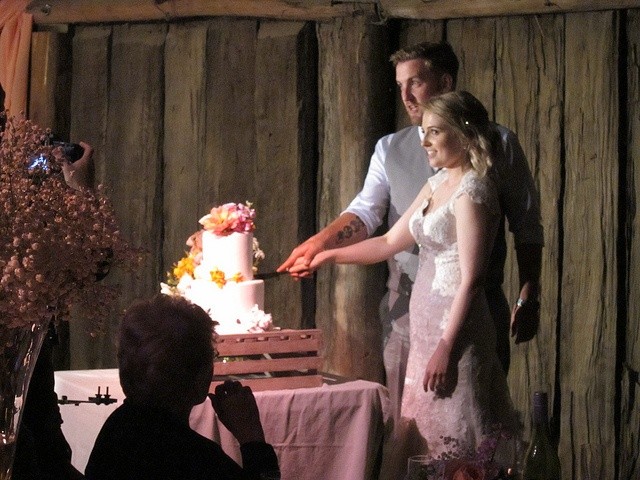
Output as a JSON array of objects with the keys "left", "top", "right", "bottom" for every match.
[{"left": 515, "top": 297, "right": 540, "bottom": 313}]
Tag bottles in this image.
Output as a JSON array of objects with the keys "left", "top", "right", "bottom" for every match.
[{"left": 521, "top": 391, "right": 563, "bottom": 480}]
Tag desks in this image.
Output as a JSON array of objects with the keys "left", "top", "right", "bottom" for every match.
[{"left": 13, "top": 368, "right": 389, "bottom": 477}]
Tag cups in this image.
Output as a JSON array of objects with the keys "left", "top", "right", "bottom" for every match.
[
  {"left": 579, "top": 441, "right": 608, "bottom": 480},
  {"left": 407, "top": 453, "right": 446, "bottom": 479}
]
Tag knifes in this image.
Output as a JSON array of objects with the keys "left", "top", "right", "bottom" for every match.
[{"left": 254, "top": 271, "right": 290, "bottom": 280}]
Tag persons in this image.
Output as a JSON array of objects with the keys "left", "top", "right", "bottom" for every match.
[
  {"left": 284, "top": 89, "right": 521, "bottom": 478},
  {"left": 275, "top": 38, "right": 545, "bottom": 478},
  {"left": 1, "top": 81, "right": 114, "bottom": 480},
  {"left": 83, "top": 292, "right": 283, "bottom": 479}
]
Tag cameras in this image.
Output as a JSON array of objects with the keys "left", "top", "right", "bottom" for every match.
[{"left": 29, "top": 134, "right": 95, "bottom": 176}]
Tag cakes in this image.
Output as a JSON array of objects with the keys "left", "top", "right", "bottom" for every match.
[{"left": 159, "top": 199, "right": 275, "bottom": 336}]
